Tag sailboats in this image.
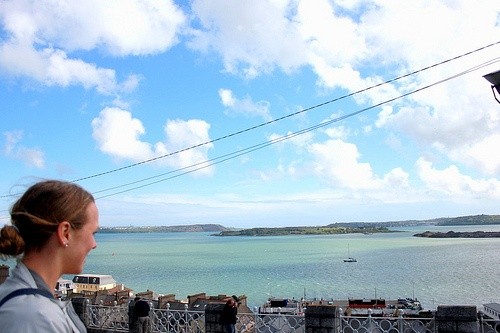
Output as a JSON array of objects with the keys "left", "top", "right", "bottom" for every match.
[{"left": 344, "top": 244, "right": 357, "bottom": 262}]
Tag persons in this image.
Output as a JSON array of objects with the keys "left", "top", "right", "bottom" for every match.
[
  {"left": 222, "top": 299, "right": 237, "bottom": 333},
  {"left": 134, "top": 297, "right": 150, "bottom": 333},
  {"left": 0, "top": 180, "right": 100, "bottom": 333}
]
